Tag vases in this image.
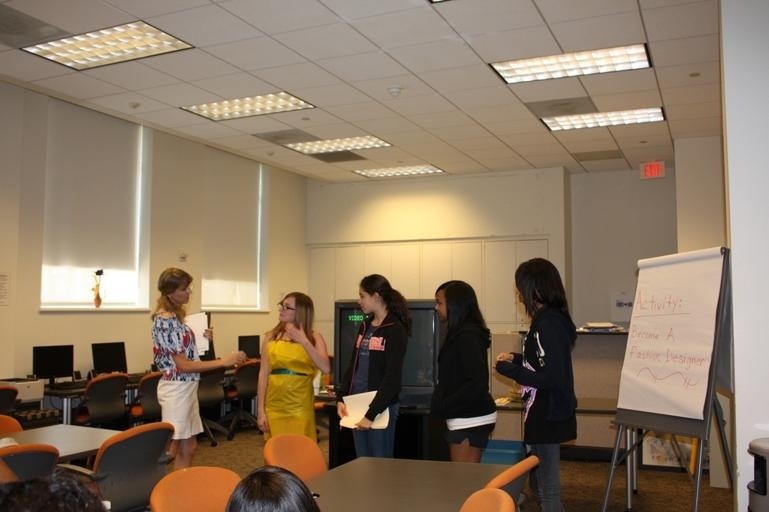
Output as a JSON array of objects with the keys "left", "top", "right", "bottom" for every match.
[{"left": 94, "top": 296, "right": 102, "bottom": 308}]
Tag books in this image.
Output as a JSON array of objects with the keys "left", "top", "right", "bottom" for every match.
[{"left": 576, "top": 322, "right": 624, "bottom": 333}]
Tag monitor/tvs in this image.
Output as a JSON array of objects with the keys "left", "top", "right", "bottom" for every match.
[
  {"left": 30, "top": 343, "right": 74, "bottom": 388},
  {"left": 237, "top": 333, "right": 261, "bottom": 359},
  {"left": 334, "top": 299, "right": 446, "bottom": 410},
  {"left": 92, "top": 341, "right": 129, "bottom": 375}
]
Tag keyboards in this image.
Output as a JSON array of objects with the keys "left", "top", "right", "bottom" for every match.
[
  {"left": 43, "top": 381, "right": 86, "bottom": 389},
  {"left": 128, "top": 377, "right": 142, "bottom": 384}
]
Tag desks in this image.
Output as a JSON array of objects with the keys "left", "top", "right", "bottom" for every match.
[
  {"left": 43, "top": 374, "right": 142, "bottom": 425},
  {"left": 491, "top": 392, "right": 638, "bottom": 510},
  {"left": 304, "top": 456, "right": 503, "bottom": 512},
  {"left": 0, "top": 424, "right": 125, "bottom": 470}
]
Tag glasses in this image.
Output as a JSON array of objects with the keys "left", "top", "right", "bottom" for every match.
[
  {"left": 312, "top": 492, "right": 320, "bottom": 499},
  {"left": 277, "top": 303, "right": 296, "bottom": 310}
]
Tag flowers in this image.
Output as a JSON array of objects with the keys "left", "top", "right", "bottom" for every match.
[{"left": 92, "top": 269, "right": 103, "bottom": 296}]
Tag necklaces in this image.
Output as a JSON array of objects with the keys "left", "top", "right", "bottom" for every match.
[{"left": 372, "top": 319, "right": 382, "bottom": 324}]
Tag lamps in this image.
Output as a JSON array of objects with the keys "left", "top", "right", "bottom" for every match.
[
  {"left": 180, "top": 91, "right": 316, "bottom": 122},
  {"left": 488, "top": 44, "right": 652, "bottom": 85},
  {"left": 19, "top": 20, "right": 193, "bottom": 72},
  {"left": 351, "top": 164, "right": 443, "bottom": 178},
  {"left": 281, "top": 136, "right": 393, "bottom": 155},
  {"left": 538, "top": 106, "right": 666, "bottom": 132}
]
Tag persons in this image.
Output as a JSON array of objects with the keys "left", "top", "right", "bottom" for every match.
[
  {"left": 225, "top": 465, "right": 321, "bottom": 512},
  {"left": 428, "top": 280, "right": 498, "bottom": 463},
  {"left": 0, "top": 468, "right": 109, "bottom": 512},
  {"left": 256, "top": 292, "right": 330, "bottom": 445},
  {"left": 494, "top": 258, "right": 578, "bottom": 512},
  {"left": 335, "top": 274, "right": 412, "bottom": 459},
  {"left": 151, "top": 267, "right": 246, "bottom": 469}
]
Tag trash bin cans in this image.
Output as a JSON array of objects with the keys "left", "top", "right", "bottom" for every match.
[{"left": 746, "top": 438, "right": 769, "bottom": 512}]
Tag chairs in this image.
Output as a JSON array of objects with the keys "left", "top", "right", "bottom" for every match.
[
  {"left": 138, "top": 372, "right": 162, "bottom": 425},
  {"left": 459, "top": 488, "right": 517, "bottom": 512},
  {"left": 0, "top": 415, "right": 24, "bottom": 436},
  {"left": 485, "top": 455, "right": 540, "bottom": 512},
  {"left": 57, "top": 422, "right": 172, "bottom": 512},
  {"left": 150, "top": 467, "right": 242, "bottom": 511},
  {"left": 216, "top": 364, "right": 264, "bottom": 437},
  {"left": 264, "top": 434, "right": 327, "bottom": 481},
  {"left": 0, "top": 387, "right": 19, "bottom": 414},
  {"left": 0, "top": 445, "right": 58, "bottom": 490},
  {"left": 73, "top": 373, "right": 128, "bottom": 427},
  {"left": 196, "top": 367, "right": 233, "bottom": 447}
]
